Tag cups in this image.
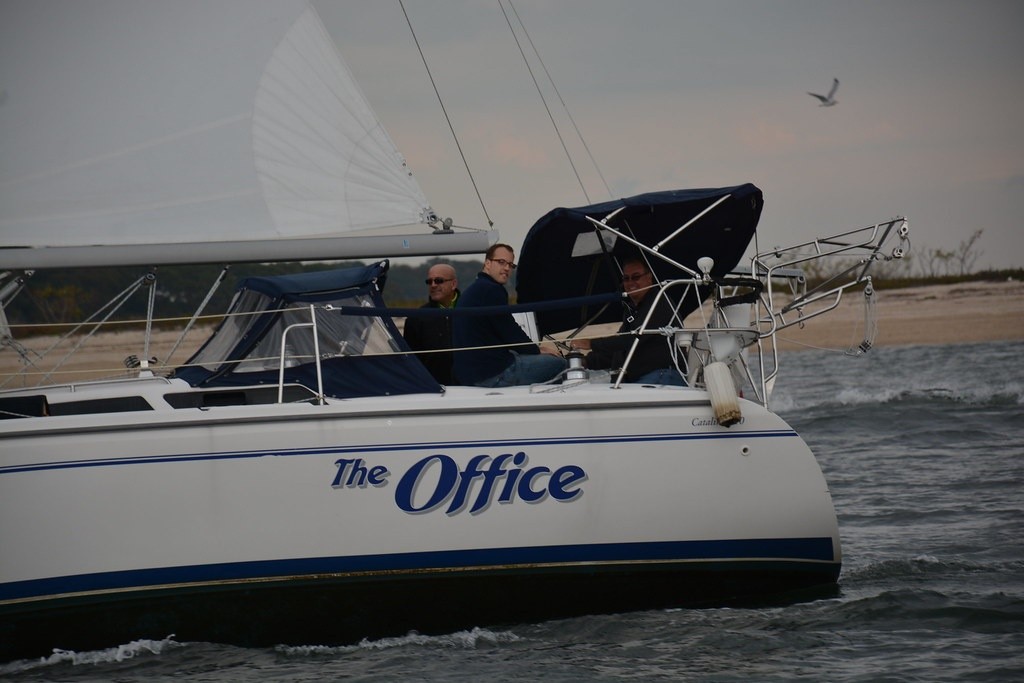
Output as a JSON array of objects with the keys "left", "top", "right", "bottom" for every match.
[{"left": 566, "top": 353, "right": 584, "bottom": 368}]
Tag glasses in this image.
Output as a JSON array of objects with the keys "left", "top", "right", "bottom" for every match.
[
  {"left": 622, "top": 271, "right": 649, "bottom": 282},
  {"left": 490, "top": 259, "right": 516, "bottom": 270},
  {"left": 425, "top": 278, "right": 453, "bottom": 286}
]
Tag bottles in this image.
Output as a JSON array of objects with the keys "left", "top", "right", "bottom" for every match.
[{"left": 284, "top": 344, "right": 297, "bottom": 368}]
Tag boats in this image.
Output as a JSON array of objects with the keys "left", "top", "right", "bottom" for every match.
[{"left": 1, "top": 183, "right": 911, "bottom": 660}]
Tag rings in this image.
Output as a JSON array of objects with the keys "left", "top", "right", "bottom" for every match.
[{"left": 573, "top": 344, "right": 576, "bottom": 346}]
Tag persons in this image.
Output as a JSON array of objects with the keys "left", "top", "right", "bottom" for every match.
[
  {"left": 570, "top": 258, "right": 688, "bottom": 387},
  {"left": 403, "top": 263, "right": 461, "bottom": 386},
  {"left": 448, "top": 244, "right": 567, "bottom": 388}
]
